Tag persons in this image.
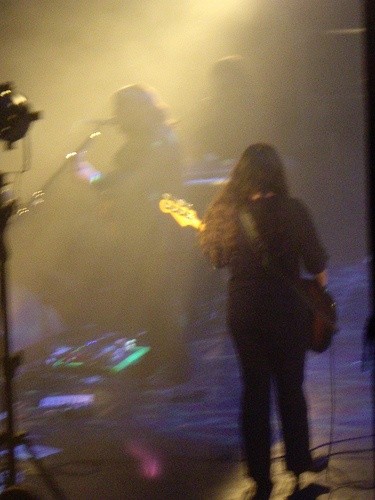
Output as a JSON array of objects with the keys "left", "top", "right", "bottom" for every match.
[
  {"left": 208, "top": 54, "right": 266, "bottom": 195},
  {"left": 203, "top": 141, "right": 331, "bottom": 496},
  {"left": 77, "top": 82, "right": 202, "bottom": 383}
]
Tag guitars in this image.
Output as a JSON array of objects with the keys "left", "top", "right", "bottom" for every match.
[{"left": 157, "top": 191, "right": 339, "bottom": 353}]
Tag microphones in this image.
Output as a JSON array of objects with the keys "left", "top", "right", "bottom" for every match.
[{"left": 97, "top": 118, "right": 118, "bottom": 126}]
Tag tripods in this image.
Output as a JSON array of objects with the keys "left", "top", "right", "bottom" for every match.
[{"left": 0, "top": 173, "right": 64, "bottom": 497}]
